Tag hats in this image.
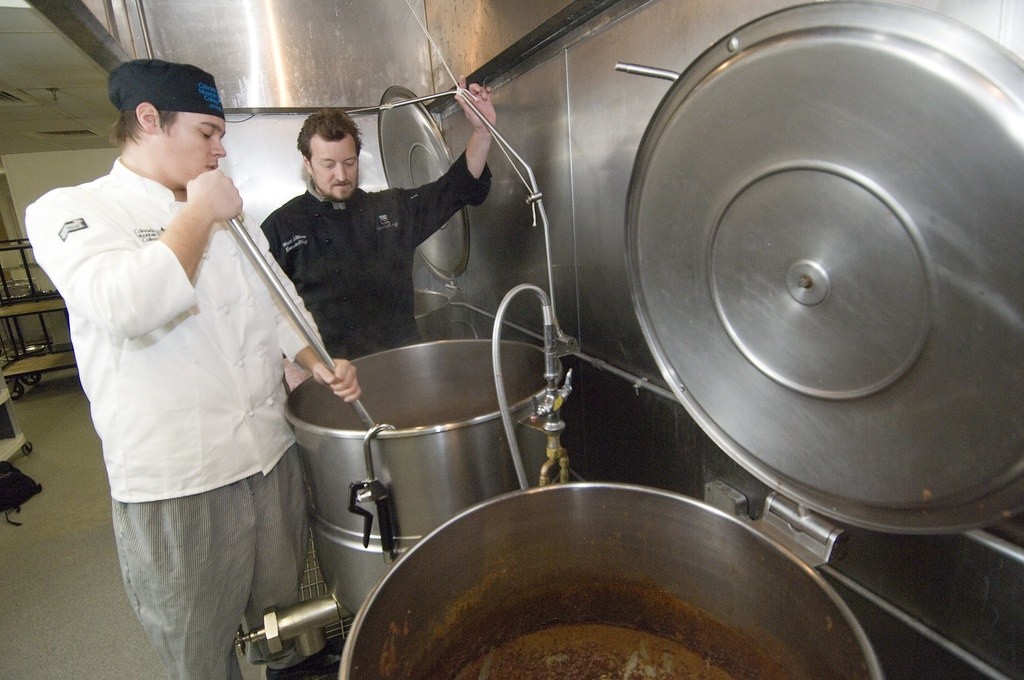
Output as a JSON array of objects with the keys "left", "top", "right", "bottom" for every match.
[{"left": 108, "top": 59, "right": 225, "bottom": 120}]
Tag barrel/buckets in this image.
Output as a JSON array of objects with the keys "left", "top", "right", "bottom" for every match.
[
  {"left": 284, "top": 338, "right": 564, "bottom": 616},
  {"left": 413, "top": 288, "right": 455, "bottom": 342},
  {"left": 337, "top": 480, "right": 886, "bottom": 680}
]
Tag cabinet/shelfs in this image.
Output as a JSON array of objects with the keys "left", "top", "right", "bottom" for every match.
[{"left": 0, "top": 238, "right": 77, "bottom": 461}]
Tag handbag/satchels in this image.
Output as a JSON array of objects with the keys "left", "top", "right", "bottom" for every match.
[{"left": 0, "top": 461, "right": 42, "bottom": 526}]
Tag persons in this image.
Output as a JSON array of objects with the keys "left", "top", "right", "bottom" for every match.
[
  {"left": 261, "top": 75, "right": 498, "bottom": 391},
  {"left": 26, "top": 58, "right": 361, "bottom": 680}
]
